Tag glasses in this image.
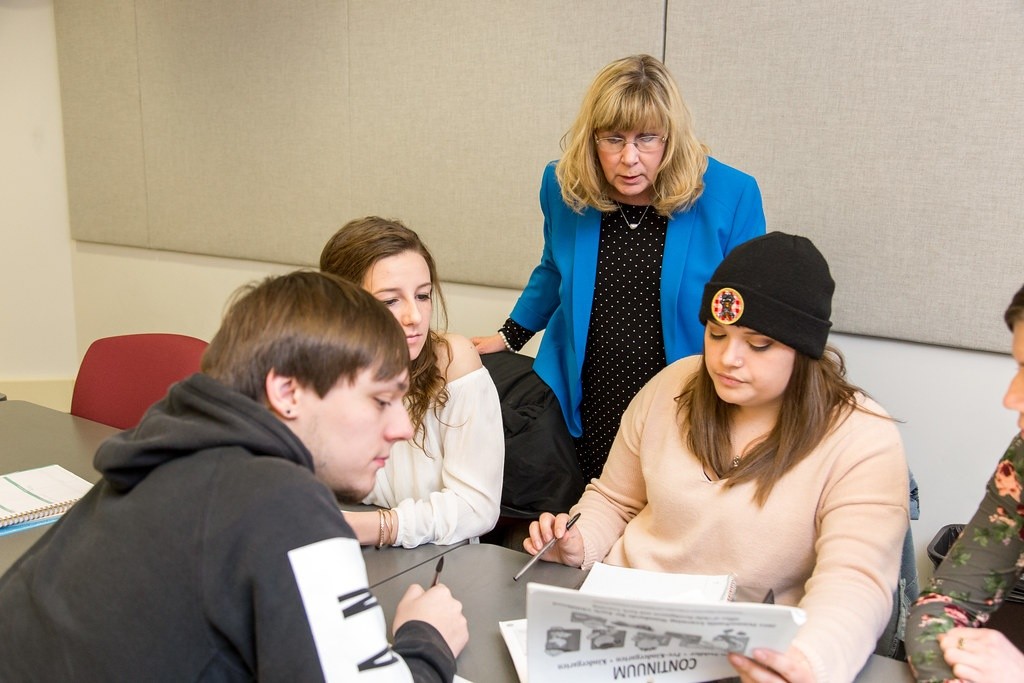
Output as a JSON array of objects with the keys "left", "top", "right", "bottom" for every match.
[{"left": 592, "top": 127, "right": 671, "bottom": 153}]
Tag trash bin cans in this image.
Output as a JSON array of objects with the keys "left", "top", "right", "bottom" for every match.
[{"left": 926, "top": 522, "right": 1024, "bottom": 658}]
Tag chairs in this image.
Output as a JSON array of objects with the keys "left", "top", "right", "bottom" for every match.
[{"left": 70, "top": 333, "right": 211, "bottom": 433}]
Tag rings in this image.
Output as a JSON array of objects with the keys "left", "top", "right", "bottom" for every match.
[{"left": 958, "top": 638, "right": 963, "bottom": 648}]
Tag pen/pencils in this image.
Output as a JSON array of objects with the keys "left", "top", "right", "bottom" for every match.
[
  {"left": 429, "top": 555, "right": 445, "bottom": 589},
  {"left": 512, "top": 513, "right": 582, "bottom": 581}
]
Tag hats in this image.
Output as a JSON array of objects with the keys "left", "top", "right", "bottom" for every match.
[{"left": 699, "top": 230, "right": 836, "bottom": 361}]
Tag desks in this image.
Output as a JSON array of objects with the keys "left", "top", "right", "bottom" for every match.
[
  {"left": 358, "top": 544, "right": 915, "bottom": 683},
  {"left": 0, "top": 399, "right": 468, "bottom": 683}
]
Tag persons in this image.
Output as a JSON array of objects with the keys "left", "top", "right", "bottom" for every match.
[
  {"left": 523, "top": 231, "right": 908, "bottom": 683},
  {"left": 0, "top": 272, "right": 470, "bottom": 683},
  {"left": 321, "top": 216, "right": 506, "bottom": 549},
  {"left": 904, "top": 284, "right": 1024, "bottom": 683},
  {"left": 470, "top": 57, "right": 767, "bottom": 484}
]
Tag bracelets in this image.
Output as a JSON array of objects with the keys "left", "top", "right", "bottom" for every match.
[
  {"left": 377, "top": 509, "right": 393, "bottom": 546},
  {"left": 499, "top": 331, "right": 518, "bottom": 353}
]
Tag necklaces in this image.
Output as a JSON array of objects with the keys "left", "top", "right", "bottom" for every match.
[
  {"left": 732, "top": 456, "right": 739, "bottom": 468},
  {"left": 614, "top": 196, "right": 651, "bottom": 230}
]
